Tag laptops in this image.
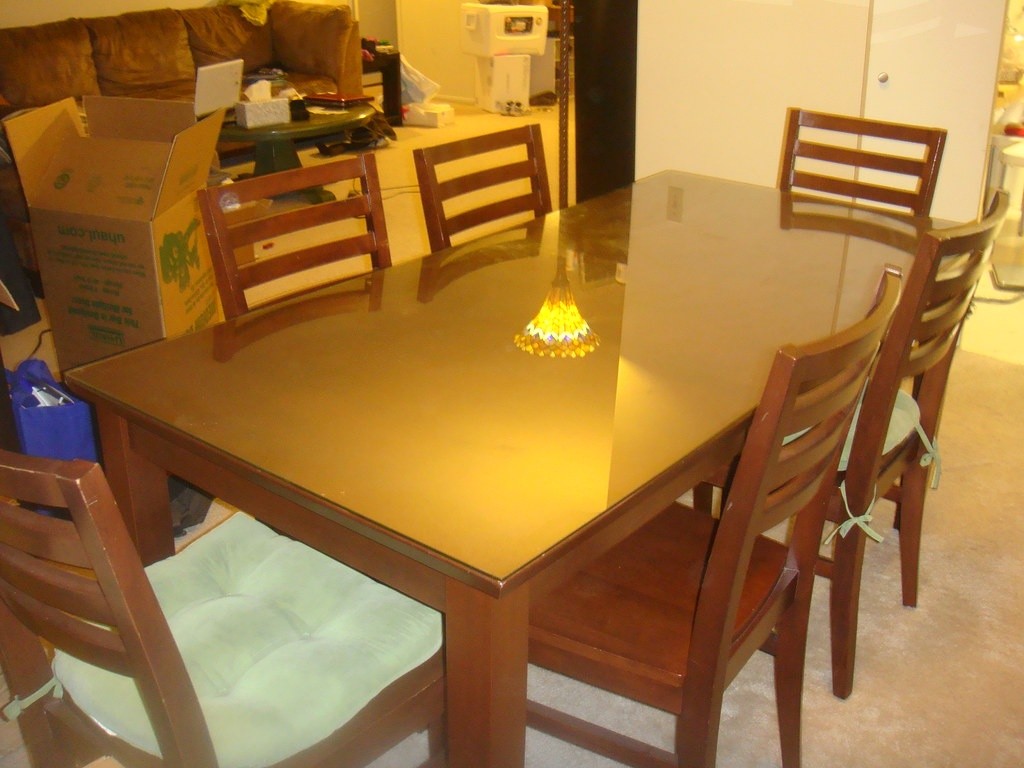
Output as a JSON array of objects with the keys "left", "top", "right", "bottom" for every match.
[{"left": 193, "top": 59, "right": 244, "bottom": 121}]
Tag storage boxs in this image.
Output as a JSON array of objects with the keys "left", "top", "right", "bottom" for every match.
[
  {"left": 1, "top": 94, "right": 230, "bottom": 366},
  {"left": 458, "top": 2, "right": 551, "bottom": 58},
  {"left": 474, "top": 54, "right": 534, "bottom": 116}
]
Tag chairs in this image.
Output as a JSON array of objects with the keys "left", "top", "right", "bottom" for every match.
[
  {"left": 690, "top": 189, "right": 1013, "bottom": 611},
  {"left": 526, "top": 260, "right": 908, "bottom": 768},
  {"left": 191, "top": 150, "right": 398, "bottom": 326},
  {"left": 0, "top": 452, "right": 446, "bottom": 768},
  {"left": 411, "top": 121, "right": 552, "bottom": 253},
  {"left": 775, "top": 108, "right": 950, "bottom": 219}
]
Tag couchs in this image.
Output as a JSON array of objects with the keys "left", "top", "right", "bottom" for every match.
[{"left": 0, "top": 2, "right": 362, "bottom": 273}]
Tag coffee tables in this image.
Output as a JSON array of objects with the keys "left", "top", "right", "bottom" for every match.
[{"left": 197, "top": 93, "right": 378, "bottom": 179}]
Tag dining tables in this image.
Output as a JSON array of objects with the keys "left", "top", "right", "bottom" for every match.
[{"left": 58, "top": 168, "right": 972, "bottom": 764}]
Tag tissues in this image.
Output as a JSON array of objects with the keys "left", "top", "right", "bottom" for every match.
[{"left": 235, "top": 79, "right": 292, "bottom": 131}]
